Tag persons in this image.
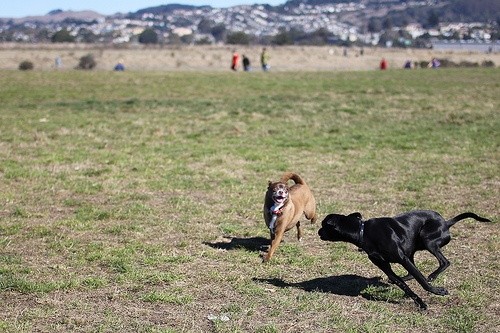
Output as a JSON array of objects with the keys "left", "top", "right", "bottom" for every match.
[
  {"left": 380, "top": 58, "right": 387, "bottom": 71},
  {"left": 426, "top": 57, "right": 440, "bottom": 68},
  {"left": 114, "top": 62, "right": 124, "bottom": 71},
  {"left": 231, "top": 50, "right": 239, "bottom": 72},
  {"left": 261, "top": 48, "right": 272, "bottom": 71},
  {"left": 405, "top": 59, "right": 420, "bottom": 69},
  {"left": 242, "top": 55, "right": 250, "bottom": 71},
  {"left": 54, "top": 54, "right": 62, "bottom": 69}
]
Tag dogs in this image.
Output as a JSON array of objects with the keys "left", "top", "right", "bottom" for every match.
[
  {"left": 319, "top": 211, "right": 491, "bottom": 310},
  {"left": 257, "top": 171, "right": 319, "bottom": 260}
]
